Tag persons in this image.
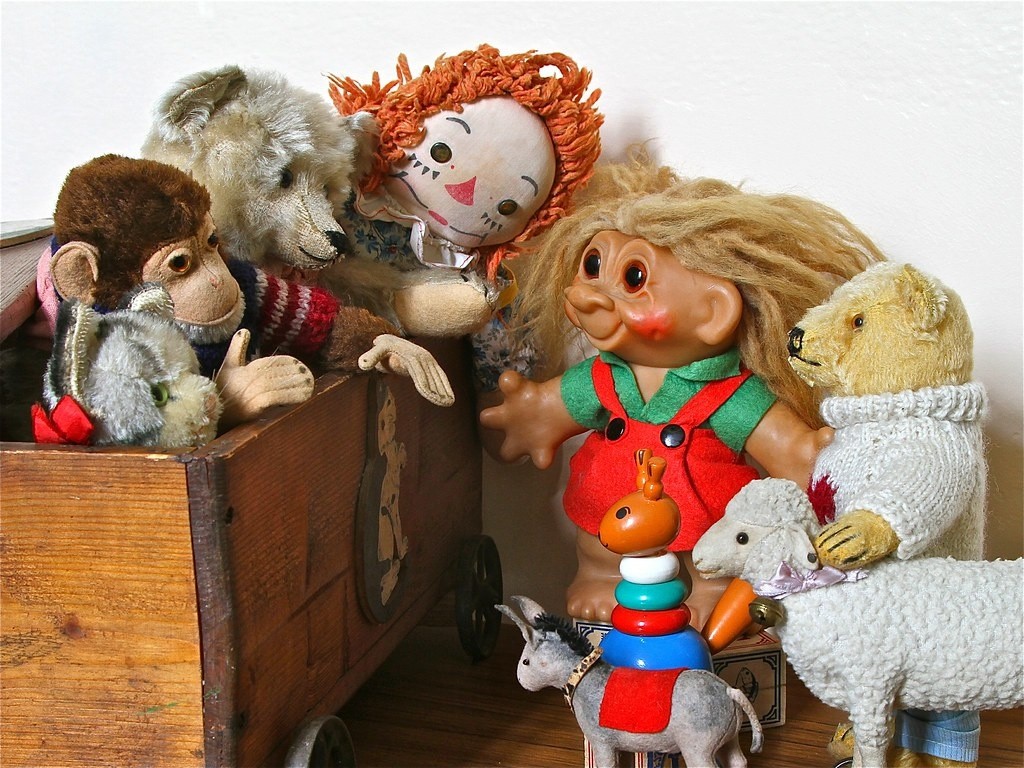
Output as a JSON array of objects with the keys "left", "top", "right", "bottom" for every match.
[{"left": 480, "top": 137, "right": 886, "bottom": 629}]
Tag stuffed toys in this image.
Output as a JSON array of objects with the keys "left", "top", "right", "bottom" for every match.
[
  {"left": 0, "top": 45, "right": 604, "bottom": 450},
  {"left": 495, "top": 260, "right": 1023, "bottom": 768}
]
